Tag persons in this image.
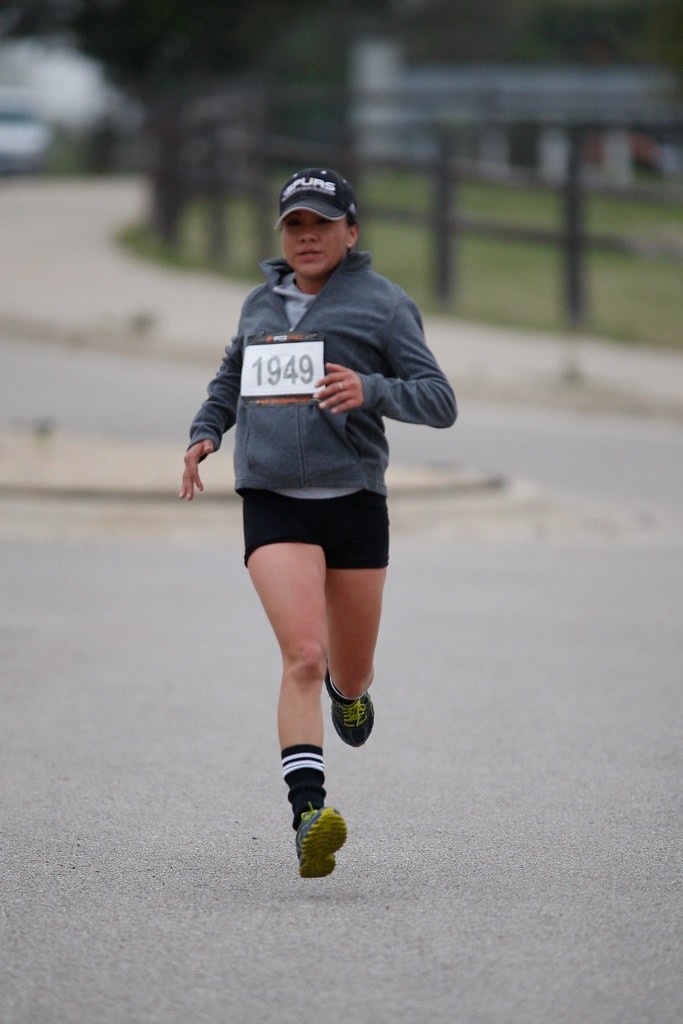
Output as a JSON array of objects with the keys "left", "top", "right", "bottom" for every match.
[{"left": 180, "top": 168, "right": 458, "bottom": 877}]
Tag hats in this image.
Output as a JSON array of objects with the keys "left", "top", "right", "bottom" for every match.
[{"left": 274, "top": 167, "right": 357, "bottom": 231}]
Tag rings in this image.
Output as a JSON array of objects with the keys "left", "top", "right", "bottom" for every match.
[{"left": 339, "top": 381, "right": 343, "bottom": 389}]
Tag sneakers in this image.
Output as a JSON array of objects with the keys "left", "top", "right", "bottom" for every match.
[
  {"left": 325, "top": 672, "right": 374, "bottom": 747},
  {"left": 295, "top": 801, "right": 348, "bottom": 878}
]
